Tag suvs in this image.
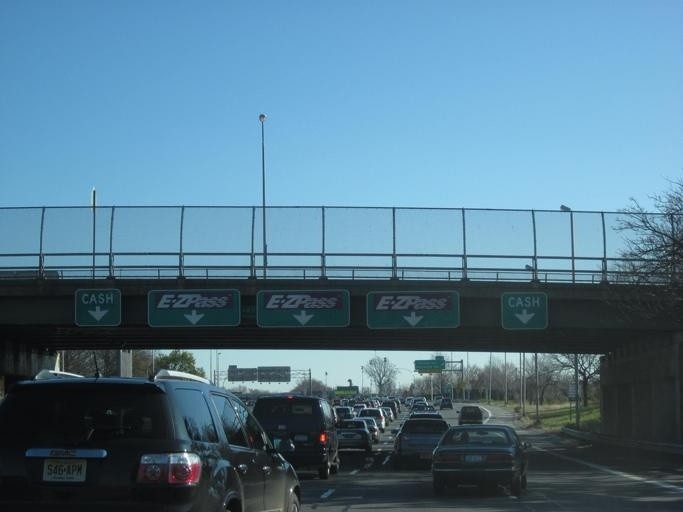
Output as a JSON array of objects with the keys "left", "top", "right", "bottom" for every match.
[
  {"left": 251, "top": 392, "right": 340, "bottom": 480},
  {"left": 0, "top": 369, "right": 302, "bottom": 512}
]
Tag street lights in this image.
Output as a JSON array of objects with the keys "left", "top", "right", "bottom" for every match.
[
  {"left": 561, "top": 205, "right": 582, "bottom": 430},
  {"left": 525, "top": 265, "right": 543, "bottom": 428}
]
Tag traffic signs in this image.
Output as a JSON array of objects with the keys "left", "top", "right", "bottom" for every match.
[
  {"left": 414, "top": 356, "right": 445, "bottom": 370},
  {"left": 258, "top": 112, "right": 268, "bottom": 278}
]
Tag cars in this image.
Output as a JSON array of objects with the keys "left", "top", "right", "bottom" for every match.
[
  {"left": 334, "top": 392, "right": 483, "bottom": 462},
  {"left": 430, "top": 424, "right": 532, "bottom": 497}
]
